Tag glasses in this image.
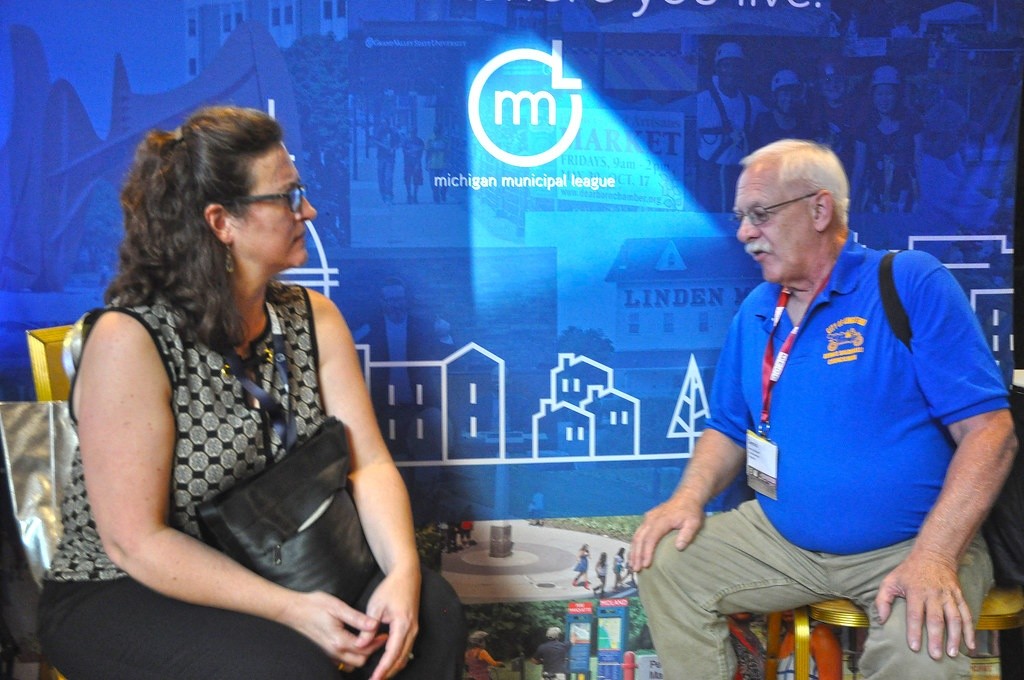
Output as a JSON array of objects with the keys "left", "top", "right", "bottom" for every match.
[
  {"left": 730, "top": 191, "right": 817, "bottom": 226},
  {"left": 199, "top": 183, "right": 306, "bottom": 215}
]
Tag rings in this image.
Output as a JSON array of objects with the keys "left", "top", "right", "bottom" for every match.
[{"left": 338, "top": 662, "right": 345, "bottom": 671}]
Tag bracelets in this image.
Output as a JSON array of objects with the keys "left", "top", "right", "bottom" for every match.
[{"left": 407, "top": 653, "right": 413, "bottom": 659}]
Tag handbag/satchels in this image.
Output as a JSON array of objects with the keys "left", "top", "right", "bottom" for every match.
[
  {"left": 880, "top": 248, "right": 1024, "bottom": 590},
  {"left": 0, "top": 309, "right": 92, "bottom": 592},
  {"left": 196, "top": 417, "right": 380, "bottom": 607}
]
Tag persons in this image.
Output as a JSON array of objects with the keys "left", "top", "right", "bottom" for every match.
[
  {"left": 628, "top": 141, "right": 1018, "bottom": 680},
  {"left": 696, "top": 18, "right": 1015, "bottom": 214},
  {"left": 370, "top": 116, "right": 447, "bottom": 204},
  {"left": 37, "top": 107, "right": 468, "bottom": 680},
  {"left": 727, "top": 609, "right": 818, "bottom": 680},
  {"left": 411, "top": 519, "right": 473, "bottom": 572},
  {"left": 465, "top": 630, "right": 504, "bottom": 680},
  {"left": 342, "top": 274, "right": 540, "bottom": 448},
  {"left": 532, "top": 627, "right": 578, "bottom": 680},
  {"left": 571, "top": 543, "right": 635, "bottom": 598}
]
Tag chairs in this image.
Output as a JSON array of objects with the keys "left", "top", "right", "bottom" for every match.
[{"left": 764, "top": 584, "right": 1024, "bottom": 680}]
